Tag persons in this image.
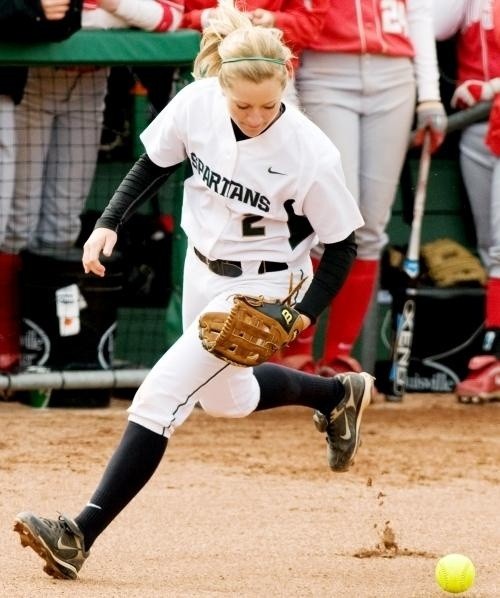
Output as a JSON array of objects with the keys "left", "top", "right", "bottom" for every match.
[{"left": 13, "top": 6, "right": 376, "bottom": 578}]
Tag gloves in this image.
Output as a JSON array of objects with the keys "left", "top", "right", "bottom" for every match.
[{"left": 414, "top": 102, "right": 450, "bottom": 155}]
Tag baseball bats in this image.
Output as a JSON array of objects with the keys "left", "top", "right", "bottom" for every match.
[{"left": 385, "top": 130, "right": 435, "bottom": 401}]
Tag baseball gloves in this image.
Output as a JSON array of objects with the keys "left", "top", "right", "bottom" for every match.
[
  {"left": 422, "top": 237, "right": 486, "bottom": 288},
  {"left": 200, "top": 270, "right": 309, "bottom": 367}
]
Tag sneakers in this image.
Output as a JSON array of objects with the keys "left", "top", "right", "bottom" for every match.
[
  {"left": 317, "top": 356, "right": 374, "bottom": 404},
  {"left": 311, "top": 371, "right": 376, "bottom": 474},
  {"left": 0, "top": 352, "right": 23, "bottom": 402},
  {"left": 455, "top": 354, "right": 500, "bottom": 405},
  {"left": 281, "top": 356, "right": 316, "bottom": 375},
  {"left": 13, "top": 509, "right": 91, "bottom": 581}
]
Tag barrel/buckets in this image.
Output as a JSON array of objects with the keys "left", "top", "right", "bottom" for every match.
[{"left": 16, "top": 246, "right": 126, "bottom": 410}]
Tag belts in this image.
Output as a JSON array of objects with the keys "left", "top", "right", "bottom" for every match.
[{"left": 194, "top": 246, "right": 289, "bottom": 278}]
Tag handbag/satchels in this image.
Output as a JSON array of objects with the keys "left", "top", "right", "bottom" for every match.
[{"left": 71, "top": 207, "right": 176, "bottom": 312}]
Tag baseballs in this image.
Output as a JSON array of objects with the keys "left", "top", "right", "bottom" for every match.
[{"left": 435, "top": 552, "right": 476, "bottom": 594}]
